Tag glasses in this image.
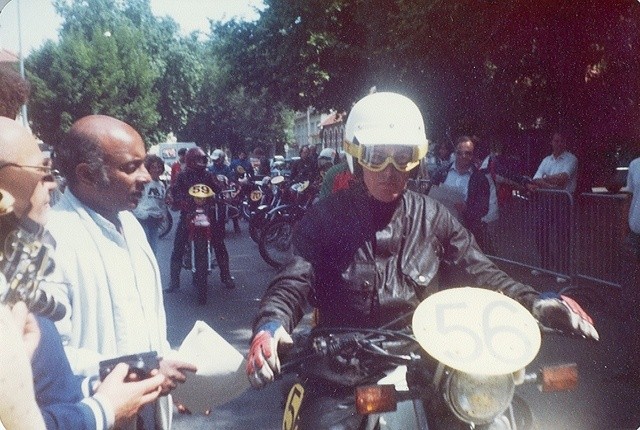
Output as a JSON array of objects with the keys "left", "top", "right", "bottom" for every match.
[
  {"left": 456, "top": 150, "right": 474, "bottom": 157},
  {"left": 342, "top": 139, "right": 428, "bottom": 173},
  {"left": 1, "top": 161, "right": 57, "bottom": 183}
]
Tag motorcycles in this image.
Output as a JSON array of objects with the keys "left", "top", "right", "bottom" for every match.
[
  {"left": 172, "top": 184, "right": 227, "bottom": 307},
  {"left": 265, "top": 287, "right": 577, "bottom": 429},
  {"left": 154, "top": 206, "right": 173, "bottom": 240},
  {"left": 224, "top": 155, "right": 321, "bottom": 270}
]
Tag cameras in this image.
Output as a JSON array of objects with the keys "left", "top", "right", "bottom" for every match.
[{"left": 0, "top": 210, "right": 68, "bottom": 322}]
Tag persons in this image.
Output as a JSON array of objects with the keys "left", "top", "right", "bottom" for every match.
[
  {"left": 206, "top": 149, "right": 241, "bottom": 235},
  {"left": 291, "top": 145, "right": 309, "bottom": 178},
  {"left": 171, "top": 148, "right": 188, "bottom": 194},
  {"left": 318, "top": 159, "right": 357, "bottom": 203},
  {"left": 246, "top": 91, "right": 600, "bottom": 429},
  {"left": 131, "top": 154, "right": 169, "bottom": 258},
  {"left": 425, "top": 136, "right": 500, "bottom": 259},
  {"left": 527, "top": 132, "right": 579, "bottom": 283},
  {"left": 230, "top": 152, "right": 254, "bottom": 179},
  {"left": 253, "top": 147, "right": 271, "bottom": 177},
  {"left": 47, "top": 115, "right": 197, "bottom": 430},
  {"left": 627, "top": 157, "right": 640, "bottom": 234},
  {"left": 164, "top": 147, "right": 235, "bottom": 294},
  {"left": 316, "top": 148, "right": 341, "bottom": 180},
  {"left": 308, "top": 144, "right": 318, "bottom": 160},
  {"left": 489, "top": 134, "right": 538, "bottom": 230},
  {"left": 0, "top": 66, "right": 165, "bottom": 430},
  {"left": 439, "top": 142, "right": 448, "bottom": 160}
]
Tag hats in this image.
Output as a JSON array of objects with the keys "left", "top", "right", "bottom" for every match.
[{"left": 210, "top": 149, "right": 225, "bottom": 160}]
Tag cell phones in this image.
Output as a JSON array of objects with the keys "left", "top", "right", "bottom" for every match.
[{"left": 98, "top": 351, "right": 160, "bottom": 382}]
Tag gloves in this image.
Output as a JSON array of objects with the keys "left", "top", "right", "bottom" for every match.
[
  {"left": 533, "top": 292, "right": 599, "bottom": 339},
  {"left": 246, "top": 320, "right": 293, "bottom": 388}
]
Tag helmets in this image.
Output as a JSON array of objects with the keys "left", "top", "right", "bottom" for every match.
[
  {"left": 343, "top": 91, "right": 426, "bottom": 183},
  {"left": 317, "top": 149, "right": 339, "bottom": 165},
  {"left": 185, "top": 147, "right": 208, "bottom": 169}
]
